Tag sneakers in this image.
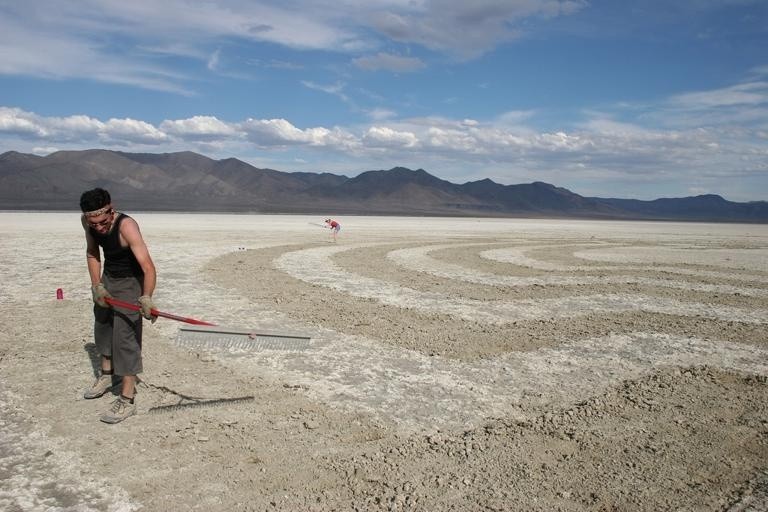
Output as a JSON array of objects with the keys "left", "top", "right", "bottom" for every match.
[
  {"left": 84, "top": 370, "right": 123, "bottom": 399},
  {"left": 100, "top": 394, "right": 137, "bottom": 424}
]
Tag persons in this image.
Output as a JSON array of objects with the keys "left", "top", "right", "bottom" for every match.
[
  {"left": 79, "top": 187, "right": 157, "bottom": 424},
  {"left": 322, "top": 218, "right": 341, "bottom": 244}
]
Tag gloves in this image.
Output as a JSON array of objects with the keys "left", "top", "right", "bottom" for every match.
[
  {"left": 90, "top": 282, "right": 115, "bottom": 309},
  {"left": 138, "top": 295, "right": 158, "bottom": 324}
]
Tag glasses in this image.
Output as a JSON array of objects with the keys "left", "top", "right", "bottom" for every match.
[{"left": 85, "top": 216, "right": 109, "bottom": 227}]
